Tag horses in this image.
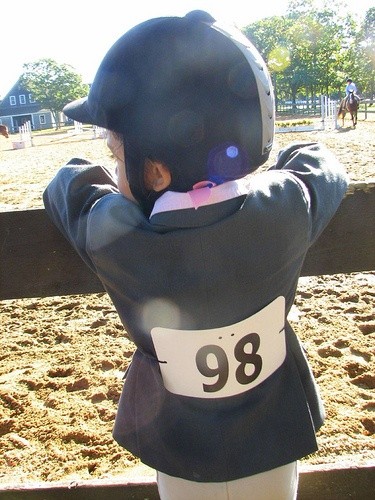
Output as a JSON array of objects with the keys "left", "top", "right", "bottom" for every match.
[
  {"left": 335, "top": 89, "right": 360, "bottom": 128},
  {"left": 0, "top": 125, "right": 9, "bottom": 138}
]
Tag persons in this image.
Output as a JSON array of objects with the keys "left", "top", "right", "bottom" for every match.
[
  {"left": 43, "top": 10, "right": 351, "bottom": 500},
  {"left": 341, "top": 79, "right": 360, "bottom": 110}
]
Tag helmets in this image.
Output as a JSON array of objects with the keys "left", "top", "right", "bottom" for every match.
[
  {"left": 62, "top": 8, "right": 274, "bottom": 178},
  {"left": 347, "top": 79, "right": 352, "bottom": 82}
]
